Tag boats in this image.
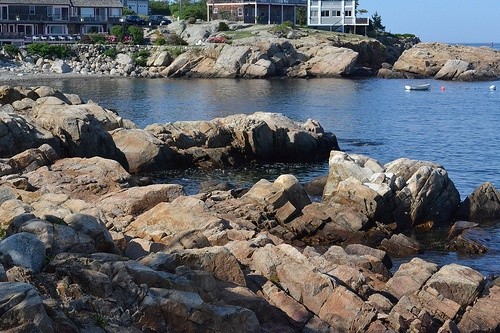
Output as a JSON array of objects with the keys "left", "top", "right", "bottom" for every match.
[{"left": 404, "top": 82, "right": 432, "bottom": 91}]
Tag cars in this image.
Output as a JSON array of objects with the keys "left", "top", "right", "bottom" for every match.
[
  {"left": 146, "top": 15, "right": 171, "bottom": 25},
  {"left": 119, "top": 15, "right": 145, "bottom": 25}
]
